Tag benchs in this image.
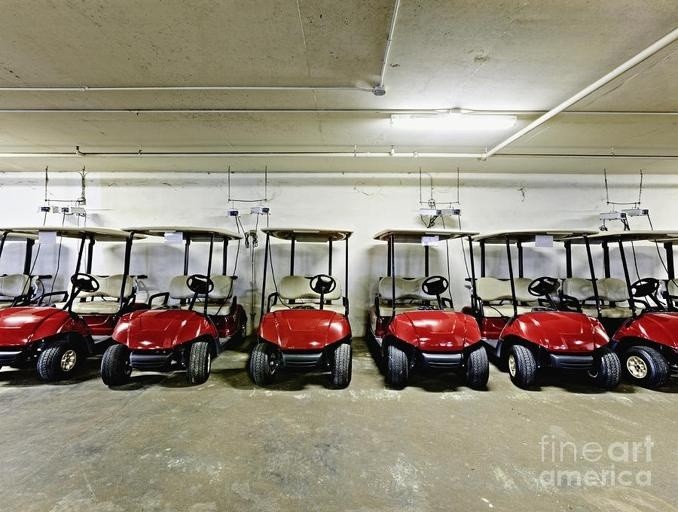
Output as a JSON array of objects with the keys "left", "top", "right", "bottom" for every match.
[
  {"left": 668, "top": 279, "right": 678, "bottom": 309},
  {"left": 563, "top": 278, "right": 642, "bottom": 318},
  {"left": 476, "top": 278, "right": 537, "bottom": 317},
  {"left": 270, "top": 276, "right": 346, "bottom": 315},
  {"left": 379, "top": 276, "right": 437, "bottom": 316},
  {"left": 169, "top": 276, "right": 233, "bottom": 315},
  {"left": 0, "top": 274, "right": 30, "bottom": 308},
  {"left": 54, "top": 274, "right": 133, "bottom": 314}
]
